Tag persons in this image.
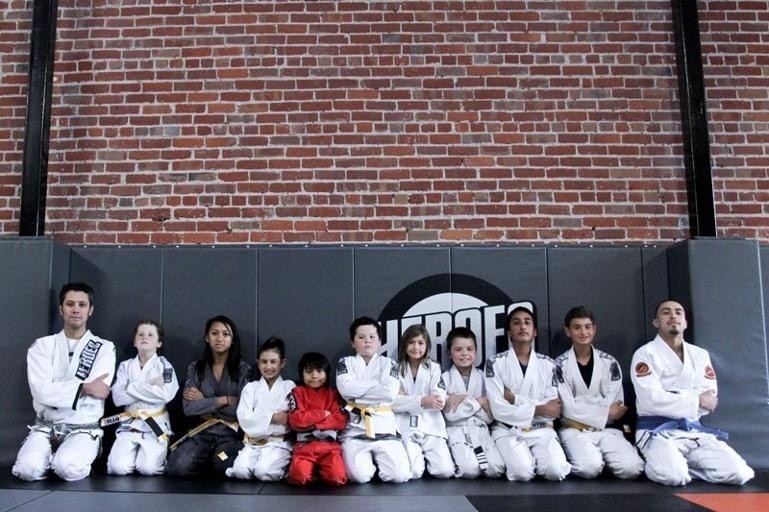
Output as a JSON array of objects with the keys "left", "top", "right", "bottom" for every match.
[
  {"left": 225, "top": 335, "right": 296, "bottom": 483},
  {"left": 287, "top": 351, "right": 348, "bottom": 488},
  {"left": 11, "top": 282, "right": 117, "bottom": 483},
  {"left": 335, "top": 316, "right": 413, "bottom": 484},
  {"left": 165, "top": 314, "right": 253, "bottom": 478},
  {"left": 439, "top": 326, "right": 506, "bottom": 480},
  {"left": 554, "top": 307, "right": 645, "bottom": 481},
  {"left": 483, "top": 306, "right": 573, "bottom": 481},
  {"left": 629, "top": 298, "right": 756, "bottom": 485},
  {"left": 391, "top": 324, "right": 456, "bottom": 479},
  {"left": 106, "top": 318, "right": 181, "bottom": 476}
]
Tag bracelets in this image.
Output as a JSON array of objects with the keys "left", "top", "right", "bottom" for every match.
[{"left": 225, "top": 395, "right": 230, "bottom": 406}]
